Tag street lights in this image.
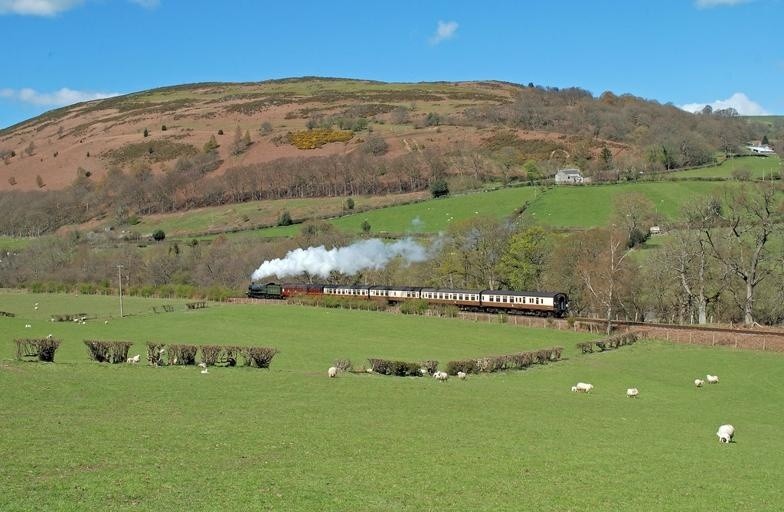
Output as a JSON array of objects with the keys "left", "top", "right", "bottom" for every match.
[{"left": 117, "top": 264, "right": 129, "bottom": 318}]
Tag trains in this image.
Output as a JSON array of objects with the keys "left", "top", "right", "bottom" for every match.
[{"left": 244, "top": 281, "right": 569, "bottom": 319}]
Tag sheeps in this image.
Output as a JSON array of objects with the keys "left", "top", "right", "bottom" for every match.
[
  {"left": 432, "top": 371, "right": 449, "bottom": 383},
  {"left": 127, "top": 354, "right": 141, "bottom": 366},
  {"left": 715, "top": 424, "right": 736, "bottom": 442},
  {"left": 200, "top": 368, "right": 209, "bottom": 374},
  {"left": 327, "top": 366, "right": 337, "bottom": 378},
  {"left": 457, "top": 370, "right": 466, "bottom": 381},
  {"left": 694, "top": 379, "right": 705, "bottom": 387},
  {"left": 572, "top": 382, "right": 594, "bottom": 394},
  {"left": 705, "top": 374, "right": 719, "bottom": 386},
  {"left": 198, "top": 362, "right": 205, "bottom": 368},
  {"left": 626, "top": 388, "right": 639, "bottom": 397}
]
